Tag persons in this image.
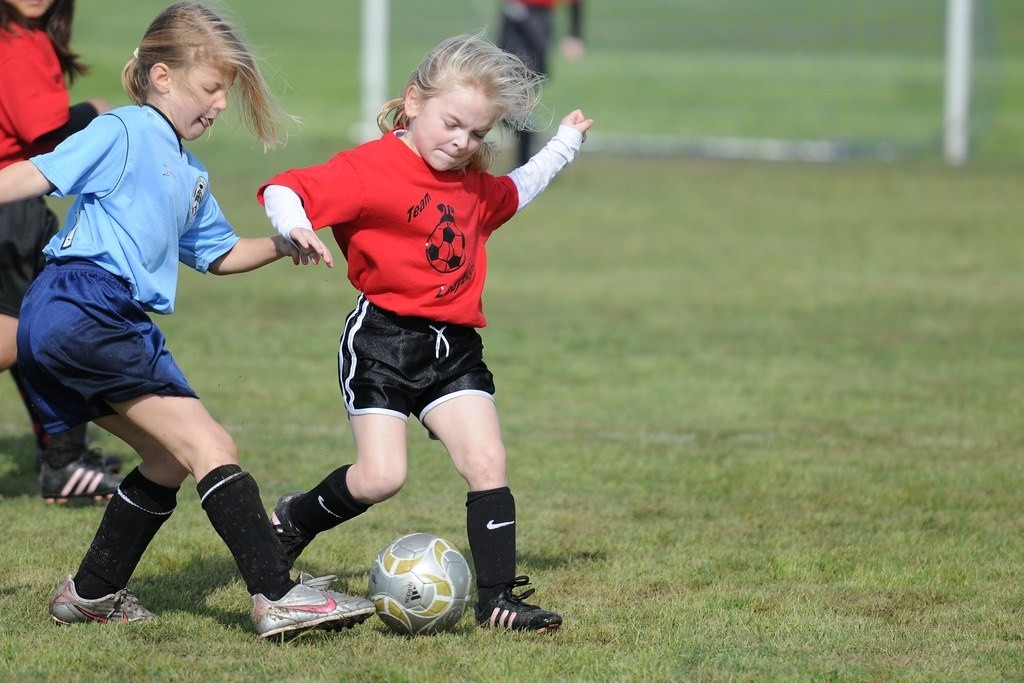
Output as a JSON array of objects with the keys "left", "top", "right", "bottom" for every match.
[
  {"left": 0, "top": 0, "right": 127, "bottom": 504},
  {"left": 257, "top": 36, "right": 595, "bottom": 633},
  {"left": 0, "top": 0, "right": 376, "bottom": 640},
  {"left": 495, "top": 0, "right": 586, "bottom": 168}
]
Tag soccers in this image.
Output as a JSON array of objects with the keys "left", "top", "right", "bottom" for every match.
[{"left": 374, "top": 533, "right": 470, "bottom": 631}]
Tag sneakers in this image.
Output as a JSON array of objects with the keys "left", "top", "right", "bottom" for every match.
[
  {"left": 250, "top": 570, "right": 376, "bottom": 639},
  {"left": 38, "top": 450, "right": 126, "bottom": 507},
  {"left": 49, "top": 574, "right": 161, "bottom": 627},
  {"left": 270, "top": 491, "right": 313, "bottom": 570},
  {"left": 473, "top": 588, "right": 563, "bottom": 634}
]
